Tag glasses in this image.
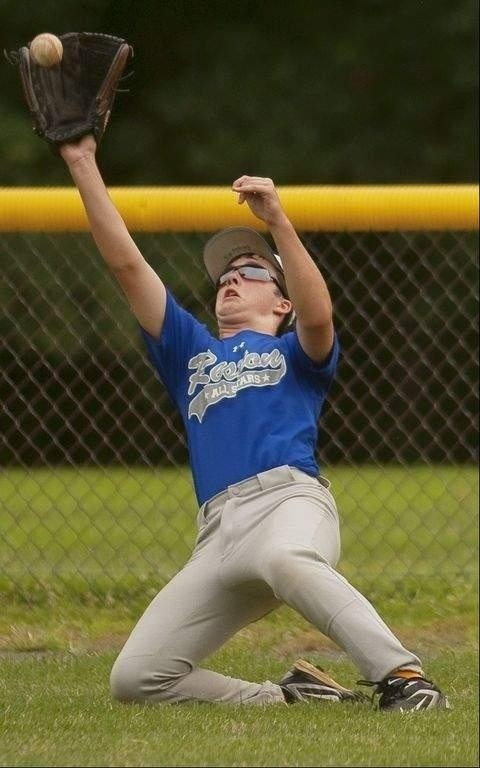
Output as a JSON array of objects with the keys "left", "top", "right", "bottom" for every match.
[{"left": 215, "top": 265, "right": 285, "bottom": 298}]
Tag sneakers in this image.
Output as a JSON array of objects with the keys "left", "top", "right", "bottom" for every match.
[
  {"left": 279, "top": 658, "right": 373, "bottom": 706},
  {"left": 379, "top": 676, "right": 443, "bottom": 710}
]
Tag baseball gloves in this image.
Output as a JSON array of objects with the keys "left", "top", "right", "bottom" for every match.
[{"left": 19, "top": 32, "right": 130, "bottom": 157}]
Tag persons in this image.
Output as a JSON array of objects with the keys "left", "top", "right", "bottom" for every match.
[{"left": 13, "top": 31, "right": 454, "bottom": 714}]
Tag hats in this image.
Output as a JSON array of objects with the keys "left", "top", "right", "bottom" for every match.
[{"left": 202, "top": 225, "right": 297, "bottom": 330}]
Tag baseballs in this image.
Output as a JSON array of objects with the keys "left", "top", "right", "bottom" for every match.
[{"left": 30, "top": 33, "right": 63, "bottom": 67}]
